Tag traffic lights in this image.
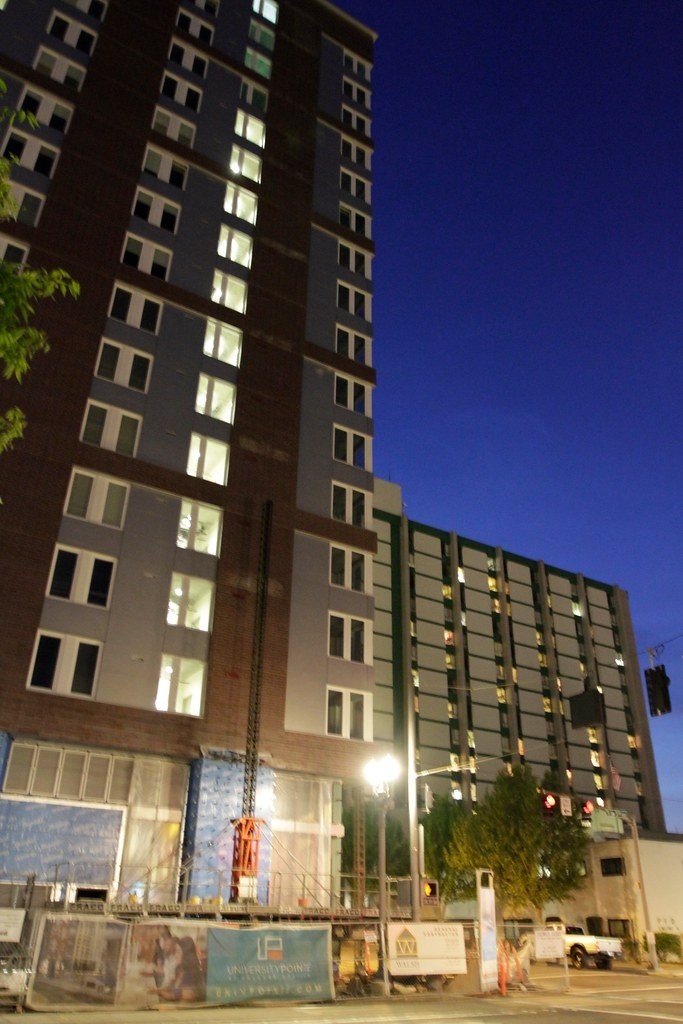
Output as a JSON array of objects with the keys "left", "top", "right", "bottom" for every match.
[
  {"left": 423, "top": 882, "right": 438, "bottom": 900},
  {"left": 579, "top": 800, "right": 594, "bottom": 820},
  {"left": 541, "top": 794, "right": 561, "bottom": 816}
]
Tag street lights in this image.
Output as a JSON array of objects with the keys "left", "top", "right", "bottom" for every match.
[{"left": 358, "top": 752, "right": 401, "bottom": 998}]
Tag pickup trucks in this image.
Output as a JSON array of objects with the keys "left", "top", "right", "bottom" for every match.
[{"left": 542, "top": 922, "right": 624, "bottom": 969}]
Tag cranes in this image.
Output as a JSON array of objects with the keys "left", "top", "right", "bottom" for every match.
[{"left": 226, "top": 495, "right": 276, "bottom": 904}]
